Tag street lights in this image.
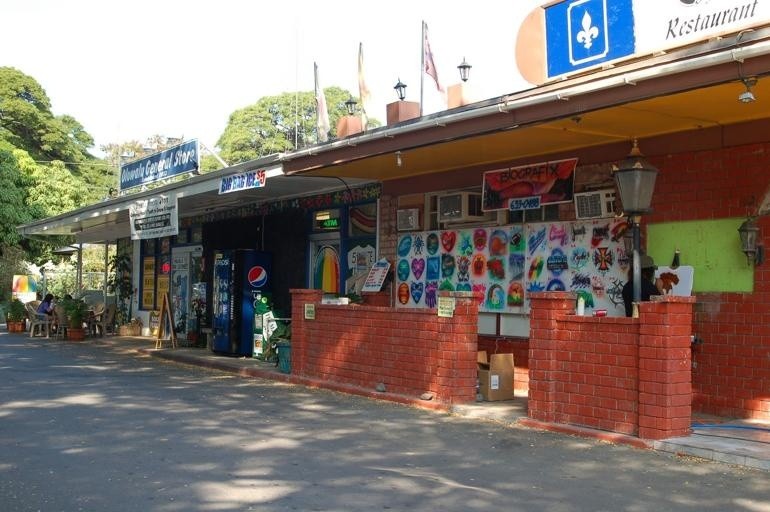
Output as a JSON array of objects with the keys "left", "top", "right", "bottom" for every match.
[{"left": 611, "top": 138, "right": 659, "bottom": 319}]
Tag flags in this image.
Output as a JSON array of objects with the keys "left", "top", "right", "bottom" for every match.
[
  {"left": 423, "top": 23, "right": 447, "bottom": 94},
  {"left": 314, "top": 66, "right": 331, "bottom": 142},
  {"left": 358, "top": 48, "right": 374, "bottom": 99}
]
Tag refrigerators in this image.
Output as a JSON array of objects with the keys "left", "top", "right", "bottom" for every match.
[{"left": 213, "top": 248, "right": 273, "bottom": 355}]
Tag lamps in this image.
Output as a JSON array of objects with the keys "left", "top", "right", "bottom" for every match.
[
  {"left": 344, "top": 93, "right": 357, "bottom": 117},
  {"left": 736, "top": 75, "right": 759, "bottom": 104},
  {"left": 457, "top": 57, "right": 471, "bottom": 82},
  {"left": 391, "top": 77, "right": 410, "bottom": 102},
  {"left": 739, "top": 205, "right": 766, "bottom": 269}
]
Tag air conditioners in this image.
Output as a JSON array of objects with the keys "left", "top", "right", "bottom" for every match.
[
  {"left": 574, "top": 187, "right": 618, "bottom": 221},
  {"left": 436, "top": 190, "right": 491, "bottom": 224}
]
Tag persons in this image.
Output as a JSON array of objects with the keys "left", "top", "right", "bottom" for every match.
[
  {"left": 622, "top": 255, "right": 665, "bottom": 319},
  {"left": 35, "top": 293, "right": 72, "bottom": 336}
]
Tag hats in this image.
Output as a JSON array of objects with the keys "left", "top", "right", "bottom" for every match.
[{"left": 638, "top": 256, "right": 658, "bottom": 270}]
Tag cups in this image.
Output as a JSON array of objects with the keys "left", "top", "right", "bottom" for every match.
[{"left": 338, "top": 298, "right": 348, "bottom": 305}]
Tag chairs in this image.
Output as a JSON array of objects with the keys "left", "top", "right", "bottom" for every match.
[{"left": 3, "top": 289, "right": 119, "bottom": 340}]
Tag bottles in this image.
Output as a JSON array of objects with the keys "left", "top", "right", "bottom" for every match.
[
  {"left": 592, "top": 310, "right": 607, "bottom": 316},
  {"left": 577, "top": 297, "right": 585, "bottom": 316}
]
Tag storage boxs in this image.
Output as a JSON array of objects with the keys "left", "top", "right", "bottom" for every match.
[
  {"left": 475, "top": 347, "right": 517, "bottom": 402},
  {"left": 119, "top": 324, "right": 141, "bottom": 336}
]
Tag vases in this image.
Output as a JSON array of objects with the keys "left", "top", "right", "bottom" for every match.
[{"left": 276, "top": 343, "right": 292, "bottom": 373}]
